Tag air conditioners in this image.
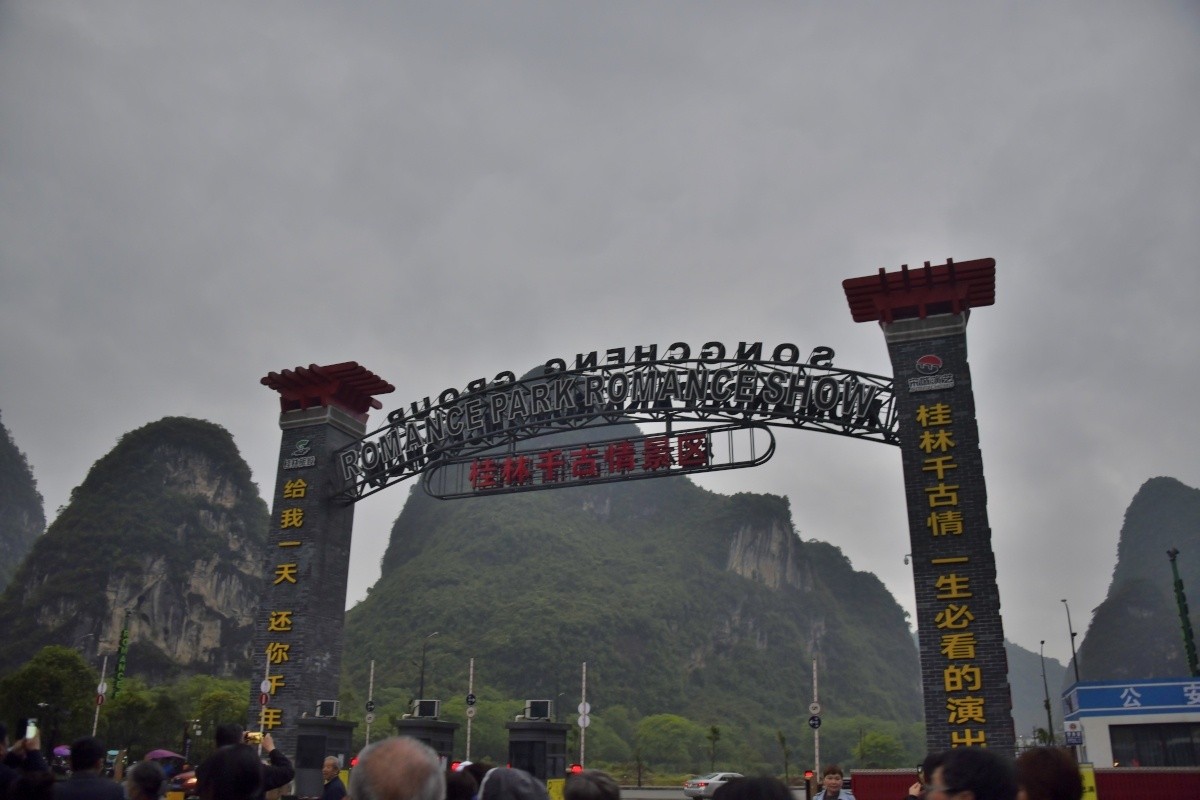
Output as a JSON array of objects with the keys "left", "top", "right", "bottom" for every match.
[
  {"left": 414, "top": 700, "right": 440, "bottom": 718},
  {"left": 315, "top": 700, "right": 340, "bottom": 717},
  {"left": 526, "top": 700, "right": 553, "bottom": 720}
]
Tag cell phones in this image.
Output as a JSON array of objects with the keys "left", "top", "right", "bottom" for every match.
[{"left": 26, "top": 717, "right": 38, "bottom": 739}]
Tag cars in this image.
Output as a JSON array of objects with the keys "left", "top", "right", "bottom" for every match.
[
  {"left": 683, "top": 770, "right": 746, "bottom": 800},
  {"left": 167, "top": 766, "right": 200, "bottom": 800}
]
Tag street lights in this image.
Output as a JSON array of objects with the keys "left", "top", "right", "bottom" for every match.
[
  {"left": 1038, "top": 640, "right": 1055, "bottom": 747},
  {"left": 1059, "top": 599, "right": 1080, "bottom": 683}
]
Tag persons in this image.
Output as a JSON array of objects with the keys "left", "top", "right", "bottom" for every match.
[
  {"left": 907, "top": 751, "right": 947, "bottom": 800},
  {"left": 347, "top": 737, "right": 447, "bottom": 800},
  {"left": 0, "top": 720, "right": 55, "bottom": 800},
  {"left": 812, "top": 765, "right": 856, "bottom": 800},
  {"left": 320, "top": 756, "right": 346, "bottom": 800},
  {"left": 125, "top": 761, "right": 164, "bottom": 800},
  {"left": 194, "top": 723, "right": 295, "bottom": 800},
  {"left": 447, "top": 761, "right": 552, "bottom": 800},
  {"left": 1016, "top": 746, "right": 1082, "bottom": 800},
  {"left": 926, "top": 746, "right": 1018, "bottom": 799},
  {"left": 563, "top": 770, "right": 621, "bottom": 800},
  {"left": 50, "top": 735, "right": 125, "bottom": 800},
  {"left": 714, "top": 775, "right": 794, "bottom": 800}
]
